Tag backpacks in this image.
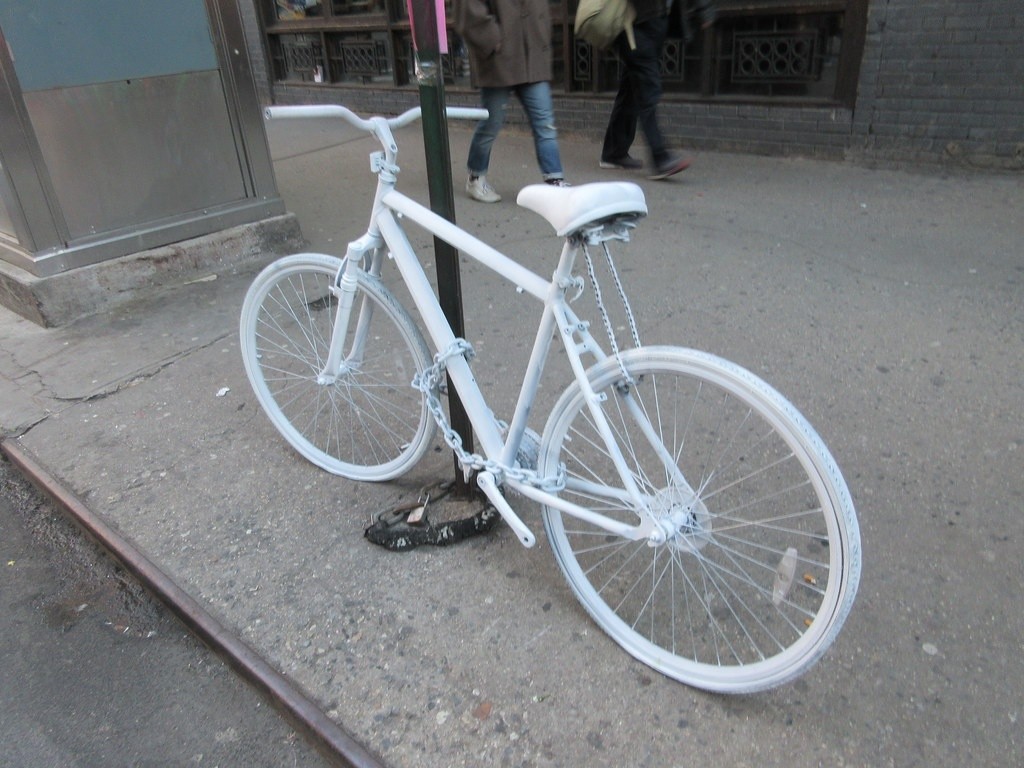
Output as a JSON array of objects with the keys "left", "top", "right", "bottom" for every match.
[{"left": 574, "top": 0, "right": 638, "bottom": 51}]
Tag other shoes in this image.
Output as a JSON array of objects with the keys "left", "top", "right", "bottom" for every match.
[
  {"left": 600, "top": 156, "right": 643, "bottom": 170},
  {"left": 646, "top": 157, "right": 692, "bottom": 179}
]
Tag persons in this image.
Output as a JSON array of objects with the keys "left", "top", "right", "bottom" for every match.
[
  {"left": 450, "top": 0, "right": 571, "bottom": 205},
  {"left": 600, "top": 0, "right": 713, "bottom": 180}
]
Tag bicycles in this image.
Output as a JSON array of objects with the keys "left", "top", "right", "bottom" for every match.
[{"left": 237, "top": 103, "right": 863, "bottom": 696}]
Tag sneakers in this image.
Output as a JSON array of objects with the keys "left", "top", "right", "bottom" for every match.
[
  {"left": 466, "top": 175, "right": 502, "bottom": 202},
  {"left": 548, "top": 180, "right": 572, "bottom": 188}
]
